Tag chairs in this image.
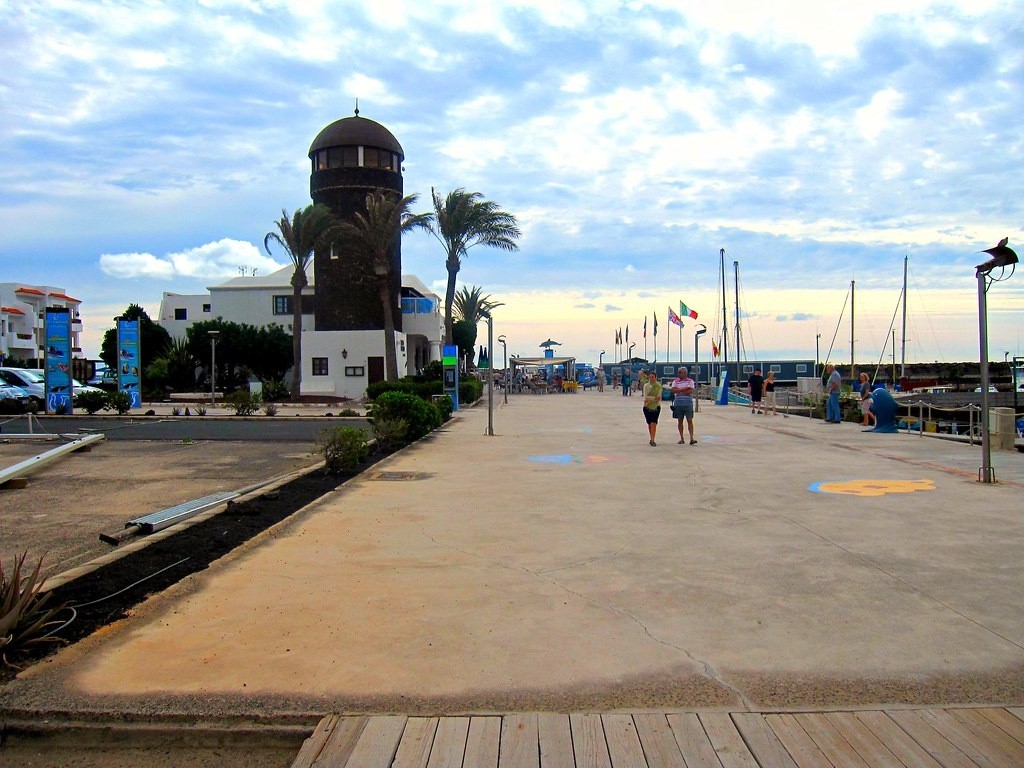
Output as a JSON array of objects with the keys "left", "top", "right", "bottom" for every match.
[{"left": 506, "top": 381, "right": 558, "bottom": 394}]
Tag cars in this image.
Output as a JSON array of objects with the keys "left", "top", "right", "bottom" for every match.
[
  {"left": 30, "top": 368, "right": 108, "bottom": 402},
  {"left": 87, "top": 367, "right": 118, "bottom": 389},
  {"left": 0, "top": 367, "right": 79, "bottom": 411},
  {"left": 0, "top": 377, "right": 32, "bottom": 415}
]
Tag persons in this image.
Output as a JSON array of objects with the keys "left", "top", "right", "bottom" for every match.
[
  {"left": 764, "top": 371, "right": 778, "bottom": 416},
  {"left": 748, "top": 369, "right": 764, "bottom": 414},
  {"left": 824, "top": 363, "right": 842, "bottom": 424},
  {"left": 493, "top": 368, "right": 647, "bottom": 396},
  {"left": 859, "top": 373, "right": 874, "bottom": 425},
  {"left": 643, "top": 373, "right": 663, "bottom": 446},
  {"left": 671, "top": 367, "right": 698, "bottom": 445}
]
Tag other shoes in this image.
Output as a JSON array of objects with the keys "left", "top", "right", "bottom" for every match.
[
  {"left": 678, "top": 441, "right": 685, "bottom": 444},
  {"left": 758, "top": 411, "right": 762, "bottom": 414},
  {"left": 824, "top": 419, "right": 841, "bottom": 424},
  {"left": 752, "top": 410, "right": 755, "bottom": 414},
  {"left": 690, "top": 440, "right": 697, "bottom": 444}
]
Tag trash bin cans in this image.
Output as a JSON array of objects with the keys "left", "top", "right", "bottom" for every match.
[{"left": 988, "top": 407, "right": 1016, "bottom": 453}]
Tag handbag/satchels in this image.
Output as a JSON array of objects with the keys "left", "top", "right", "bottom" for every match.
[{"left": 670, "top": 406, "right": 674, "bottom": 411}]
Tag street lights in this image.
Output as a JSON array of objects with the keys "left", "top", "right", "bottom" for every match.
[
  {"left": 477, "top": 307, "right": 494, "bottom": 435},
  {"left": 599, "top": 350, "right": 605, "bottom": 368},
  {"left": 498, "top": 335, "right": 508, "bottom": 404},
  {"left": 693, "top": 323, "right": 708, "bottom": 412},
  {"left": 208, "top": 331, "right": 220, "bottom": 409},
  {"left": 973, "top": 237, "right": 1019, "bottom": 486},
  {"left": 628, "top": 342, "right": 636, "bottom": 397}
]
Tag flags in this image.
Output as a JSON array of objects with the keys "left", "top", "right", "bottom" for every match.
[
  {"left": 669, "top": 308, "right": 685, "bottom": 328},
  {"left": 653, "top": 313, "right": 659, "bottom": 336},
  {"left": 644, "top": 317, "right": 647, "bottom": 338},
  {"left": 615, "top": 326, "right": 628, "bottom": 345},
  {"left": 680, "top": 301, "right": 699, "bottom": 321}
]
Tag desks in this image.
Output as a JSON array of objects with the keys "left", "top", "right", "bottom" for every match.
[
  {"left": 562, "top": 381, "right": 574, "bottom": 393},
  {"left": 535, "top": 382, "right": 547, "bottom": 394}
]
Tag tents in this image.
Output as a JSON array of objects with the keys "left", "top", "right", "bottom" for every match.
[{"left": 509, "top": 357, "right": 575, "bottom": 395}]
